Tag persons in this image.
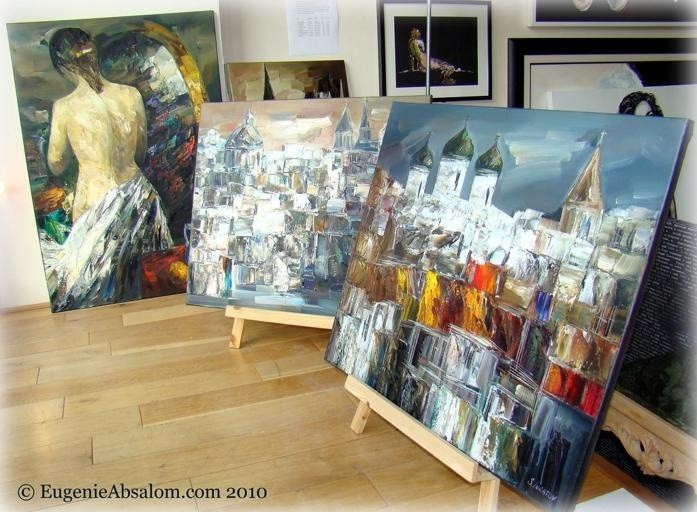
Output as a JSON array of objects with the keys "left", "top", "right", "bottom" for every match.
[
  {"left": 47, "top": 27, "right": 174, "bottom": 314},
  {"left": 408, "top": 27, "right": 456, "bottom": 86},
  {"left": 618, "top": 91, "right": 678, "bottom": 218}
]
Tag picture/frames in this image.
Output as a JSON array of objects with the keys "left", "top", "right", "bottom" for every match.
[
  {"left": 378, "top": 0, "right": 492, "bottom": 101},
  {"left": 528, "top": 0, "right": 697, "bottom": 30},
  {"left": 506, "top": 38, "right": 697, "bottom": 109}
]
